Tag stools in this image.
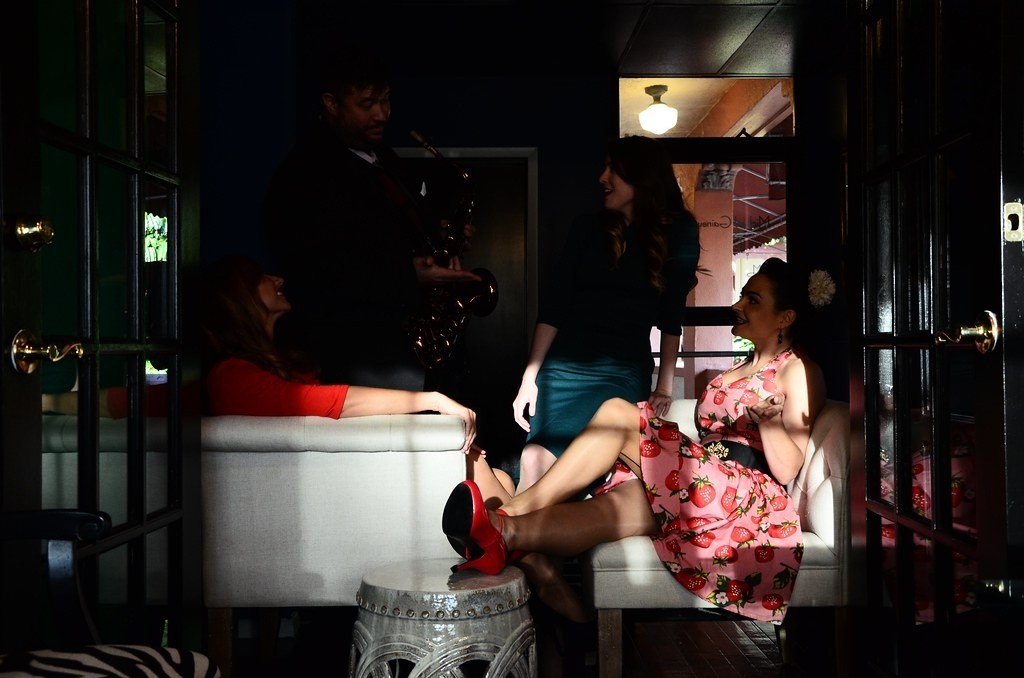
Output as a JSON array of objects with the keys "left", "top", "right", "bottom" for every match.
[{"left": 350, "top": 558, "right": 537, "bottom": 678}]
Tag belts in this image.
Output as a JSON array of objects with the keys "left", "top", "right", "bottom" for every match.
[{"left": 704, "top": 440, "right": 776, "bottom": 479}]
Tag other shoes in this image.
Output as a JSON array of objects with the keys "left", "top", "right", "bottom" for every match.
[{"left": 534, "top": 591, "right": 597, "bottom": 636}]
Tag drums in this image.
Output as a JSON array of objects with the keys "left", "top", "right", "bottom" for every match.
[{"left": 339, "top": 554, "right": 551, "bottom": 678}]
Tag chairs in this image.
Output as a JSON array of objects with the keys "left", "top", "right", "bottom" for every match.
[
  {"left": 41, "top": 414, "right": 169, "bottom": 604},
  {"left": 201, "top": 414, "right": 468, "bottom": 678},
  {"left": 590, "top": 401, "right": 851, "bottom": 678}
]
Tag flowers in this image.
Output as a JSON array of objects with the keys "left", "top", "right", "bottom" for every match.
[{"left": 808, "top": 269, "right": 838, "bottom": 308}]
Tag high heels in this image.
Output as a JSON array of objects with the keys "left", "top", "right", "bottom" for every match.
[{"left": 442, "top": 480, "right": 527, "bottom": 576}]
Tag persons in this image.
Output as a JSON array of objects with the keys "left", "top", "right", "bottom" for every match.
[
  {"left": 200, "top": 260, "right": 636, "bottom": 656},
  {"left": 278, "top": 61, "right": 482, "bottom": 389},
  {"left": 443, "top": 257, "right": 839, "bottom": 626},
  {"left": 512, "top": 134, "right": 700, "bottom": 678}
]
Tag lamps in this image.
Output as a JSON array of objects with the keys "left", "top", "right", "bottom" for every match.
[{"left": 639, "top": 84, "right": 678, "bottom": 135}]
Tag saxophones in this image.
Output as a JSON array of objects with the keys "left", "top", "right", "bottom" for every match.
[{"left": 387, "top": 111, "right": 507, "bottom": 385}]
